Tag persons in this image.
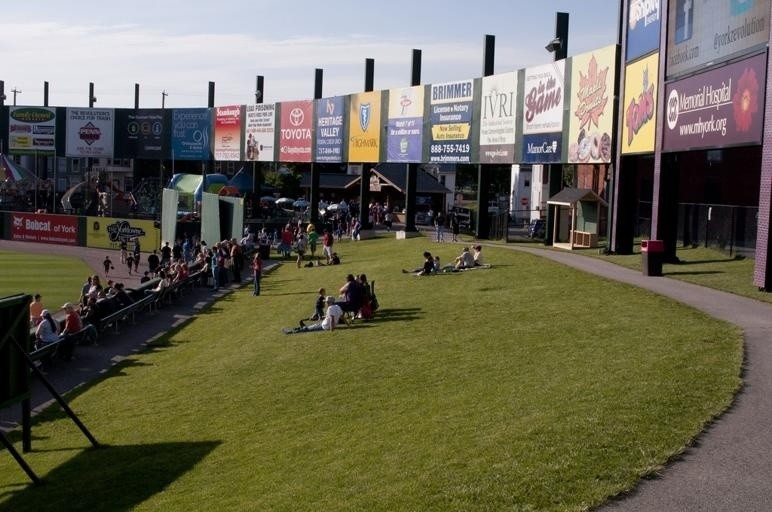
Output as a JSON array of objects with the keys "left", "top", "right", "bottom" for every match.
[
  {"left": 243, "top": 214, "right": 341, "bottom": 268},
  {"left": 449, "top": 210, "right": 460, "bottom": 243},
  {"left": 281, "top": 274, "right": 379, "bottom": 334},
  {"left": 369, "top": 200, "right": 393, "bottom": 231},
  {"left": 319, "top": 199, "right": 362, "bottom": 241},
  {"left": 120, "top": 237, "right": 140, "bottom": 275},
  {"left": 141, "top": 237, "right": 241, "bottom": 311},
  {"left": 431, "top": 255, "right": 440, "bottom": 273},
  {"left": 250, "top": 252, "right": 263, "bottom": 296},
  {"left": 34, "top": 309, "right": 61, "bottom": 346},
  {"left": 103, "top": 256, "right": 112, "bottom": 278},
  {"left": 471, "top": 245, "right": 483, "bottom": 267},
  {"left": 435, "top": 210, "right": 444, "bottom": 244},
  {"left": 30, "top": 294, "right": 46, "bottom": 327},
  {"left": 402, "top": 251, "right": 436, "bottom": 276},
  {"left": 80, "top": 274, "right": 131, "bottom": 345},
  {"left": 59, "top": 302, "right": 82, "bottom": 336},
  {"left": 455, "top": 246, "right": 475, "bottom": 269}
]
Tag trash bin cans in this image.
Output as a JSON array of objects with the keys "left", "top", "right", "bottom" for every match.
[{"left": 641, "top": 239, "right": 663, "bottom": 276}]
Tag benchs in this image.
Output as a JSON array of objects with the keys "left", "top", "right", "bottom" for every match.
[{"left": 29, "top": 259, "right": 212, "bottom": 373}]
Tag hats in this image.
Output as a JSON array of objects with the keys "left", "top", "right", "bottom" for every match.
[
  {"left": 322, "top": 295, "right": 336, "bottom": 302},
  {"left": 40, "top": 309, "right": 49, "bottom": 317},
  {"left": 60, "top": 302, "right": 74, "bottom": 309}
]
